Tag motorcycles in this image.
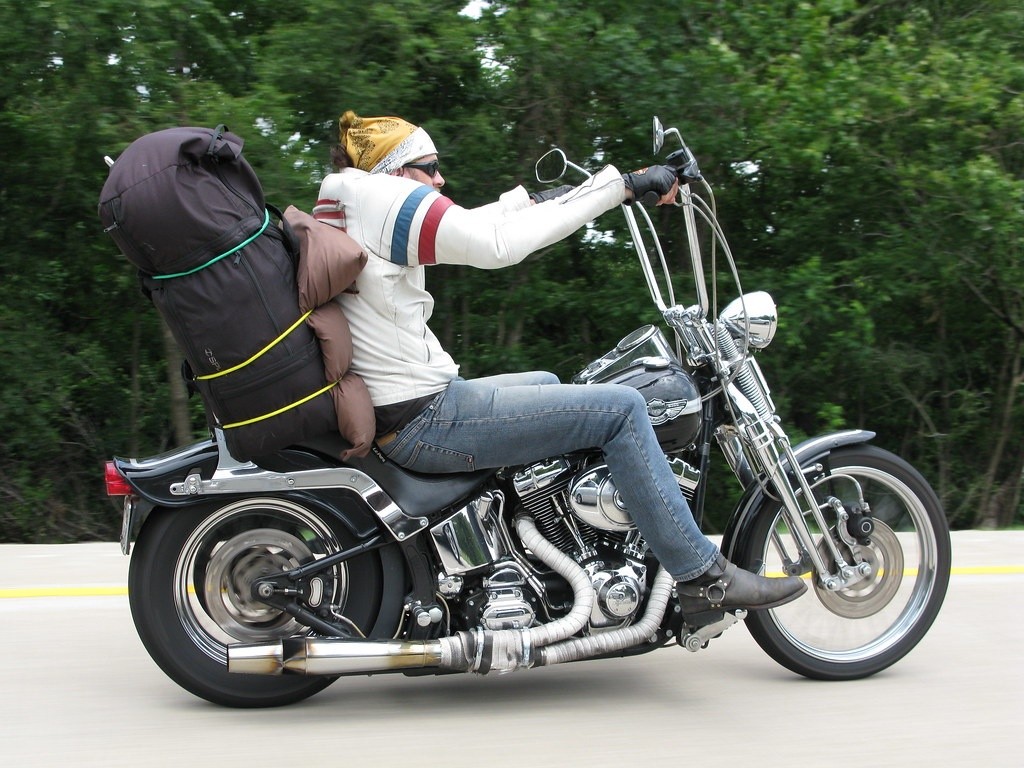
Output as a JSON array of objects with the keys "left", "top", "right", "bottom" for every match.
[{"left": 105, "top": 114, "right": 953, "bottom": 710}]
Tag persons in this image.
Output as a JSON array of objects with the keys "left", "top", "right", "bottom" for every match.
[{"left": 311, "top": 110, "right": 807, "bottom": 631}]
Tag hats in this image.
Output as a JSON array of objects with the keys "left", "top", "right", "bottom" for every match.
[{"left": 332, "top": 109, "right": 439, "bottom": 175}]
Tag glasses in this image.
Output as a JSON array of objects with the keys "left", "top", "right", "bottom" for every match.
[{"left": 402, "top": 159, "right": 439, "bottom": 179}]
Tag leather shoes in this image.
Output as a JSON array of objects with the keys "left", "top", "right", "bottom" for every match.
[{"left": 675, "top": 552, "right": 809, "bottom": 628}]
